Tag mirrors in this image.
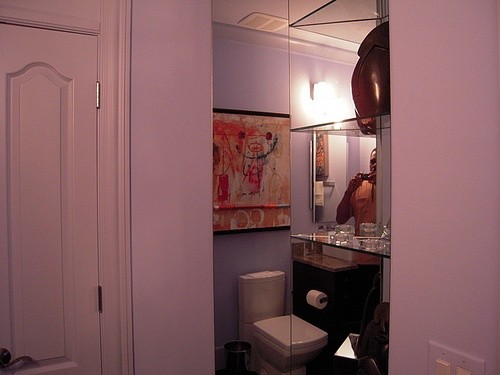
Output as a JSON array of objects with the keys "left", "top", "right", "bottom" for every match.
[{"left": 314, "top": 129, "right": 377, "bottom": 238}]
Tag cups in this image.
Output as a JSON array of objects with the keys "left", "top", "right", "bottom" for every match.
[
  {"left": 334, "top": 224, "right": 355, "bottom": 247},
  {"left": 359, "top": 222, "right": 377, "bottom": 237}
]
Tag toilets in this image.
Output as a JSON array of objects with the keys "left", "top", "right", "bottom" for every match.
[{"left": 239, "top": 271, "right": 328, "bottom": 375}]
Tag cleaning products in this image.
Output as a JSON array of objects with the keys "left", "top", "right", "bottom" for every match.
[{"left": 306, "top": 289, "right": 328, "bottom": 309}]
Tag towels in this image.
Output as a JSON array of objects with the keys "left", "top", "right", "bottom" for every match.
[{"left": 314, "top": 181, "right": 324, "bottom": 207}]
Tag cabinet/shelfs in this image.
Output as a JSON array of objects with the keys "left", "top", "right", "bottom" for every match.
[{"left": 323, "top": 182, "right": 335, "bottom": 197}]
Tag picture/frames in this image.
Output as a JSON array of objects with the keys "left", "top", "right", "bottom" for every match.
[
  {"left": 213, "top": 108, "right": 291, "bottom": 235},
  {"left": 316, "top": 134, "right": 329, "bottom": 177}
]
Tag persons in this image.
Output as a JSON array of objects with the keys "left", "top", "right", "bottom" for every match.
[{"left": 336, "top": 148, "right": 377, "bottom": 236}]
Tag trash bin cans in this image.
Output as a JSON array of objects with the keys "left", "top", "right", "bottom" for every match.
[{"left": 224, "top": 340, "right": 252, "bottom": 375}]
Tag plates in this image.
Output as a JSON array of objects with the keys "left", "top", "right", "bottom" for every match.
[{"left": 359, "top": 239, "right": 387, "bottom": 251}]
